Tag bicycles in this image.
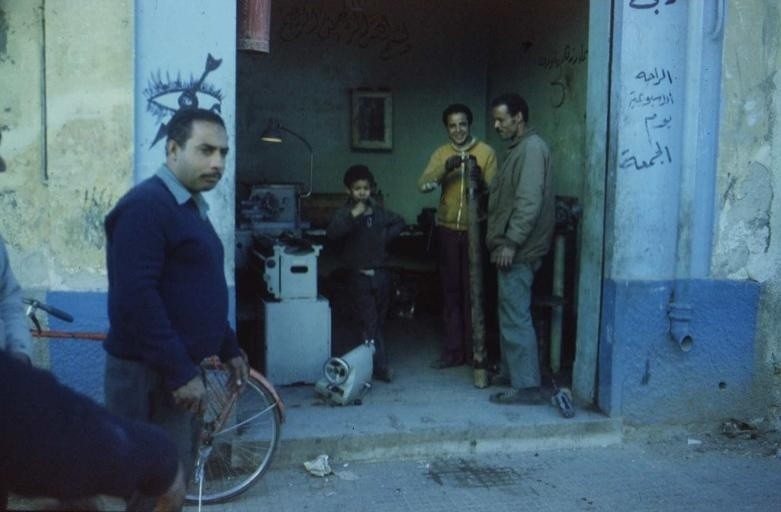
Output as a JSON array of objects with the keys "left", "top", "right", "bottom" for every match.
[{"left": 23, "top": 300, "right": 285, "bottom": 511}]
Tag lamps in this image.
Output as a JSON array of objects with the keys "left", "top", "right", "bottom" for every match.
[{"left": 260, "top": 117, "right": 314, "bottom": 198}]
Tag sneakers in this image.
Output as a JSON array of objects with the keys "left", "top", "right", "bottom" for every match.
[
  {"left": 430, "top": 359, "right": 448, "bottom": 369},
  {"left": 489, "top": 388, "right": 544, "bottom": 405}
]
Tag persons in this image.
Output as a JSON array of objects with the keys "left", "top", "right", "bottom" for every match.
[
  {"left": 1, "top": 348, "right": 187, "bottom": 512},
  {"left": 324, "top": 164, "right": 407, "bottom": 383},
  {"left": 487, "top": 94, "right": 555, "bottom": 406},
  {"left": 416, "top": 102, "right": 498, "bottom": 371},
  {"left": 1, "top": 235, "right": 34, "bottom": 363},
  {"left": 102, "top": 105, "right": 252, "bottom": 511}
]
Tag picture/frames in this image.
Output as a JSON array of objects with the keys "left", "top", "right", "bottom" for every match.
[{"left": 349, "top": 86, "right": 396, "bottom": 153}]
